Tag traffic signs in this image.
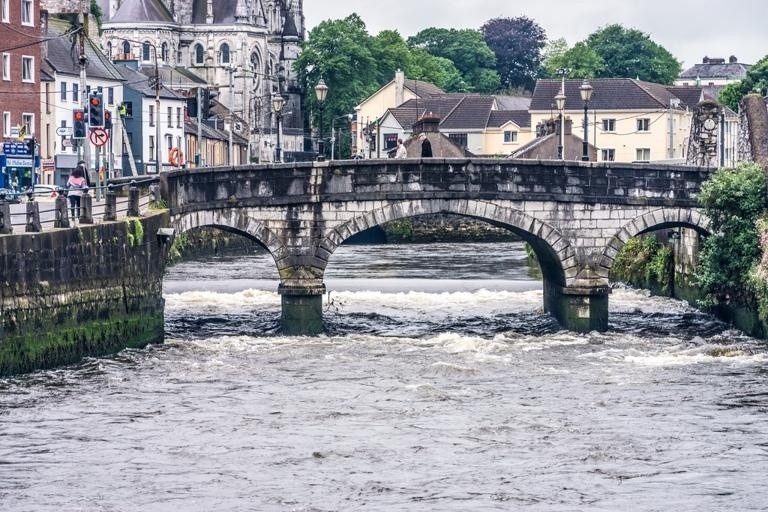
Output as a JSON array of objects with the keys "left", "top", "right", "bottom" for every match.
[
  {"left": 57, "top": 128, "right": 73, "bottom": 135},
  {"left": 62, "top": 139, "right": 81, "bottom": 146}
]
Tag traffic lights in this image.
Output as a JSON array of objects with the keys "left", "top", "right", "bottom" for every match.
[
  {"left": 90, "top": 94, "right": 103, "bottom": 126},
  {"left": 105, "top": 111, "right": 112, "bottom": 128},
  {"left": 72, "top": 109, "right": 85, "bottom": 137},
  {"left": 206, "top": 90, "right": 217, "bottom": 118}
]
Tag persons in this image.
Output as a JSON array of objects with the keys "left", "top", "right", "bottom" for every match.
[
  {"left": 418, "top": 133, "right": 432, "bottom": 157},
  {"left": 395, "top": 139, "right": 408, "bottom": 159},
  {"left": 67, "top": 167, "right": 86, "bottom": 220},
  {"left": 77, "top": 160, "right": 90, "bottom": 193}
]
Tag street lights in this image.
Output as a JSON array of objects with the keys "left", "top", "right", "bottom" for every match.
[
  {"left": 556, "top": 66, "right": 570, "bottom": 160},
  {"left": 330, "top": 114, "right": 354, "bottom": 160},
  {"left": 578, "top": 76, "right": 594, "bottom": 161},
  {"left": 273, "top": 89, "right": 284, "bottom": 163},
  {"left": 553, "top": 87, "right": 566, "bottom": 158},
  {"left": 242, "top": 68, "right": 280, "bottom": 92},
  {"left": 104, "top": 34, "right": 162, "bottom": 174},
  {"left": 314, "top": 75, "right": 329, "bottom": 161}
]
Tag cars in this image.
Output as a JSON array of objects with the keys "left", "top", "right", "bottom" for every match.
[
  {"left": 21, "top": 183, "right": 61, "bottom": 197},
  {"left": 0, "top": 188, "right": 22, "bottom": 204}
]
